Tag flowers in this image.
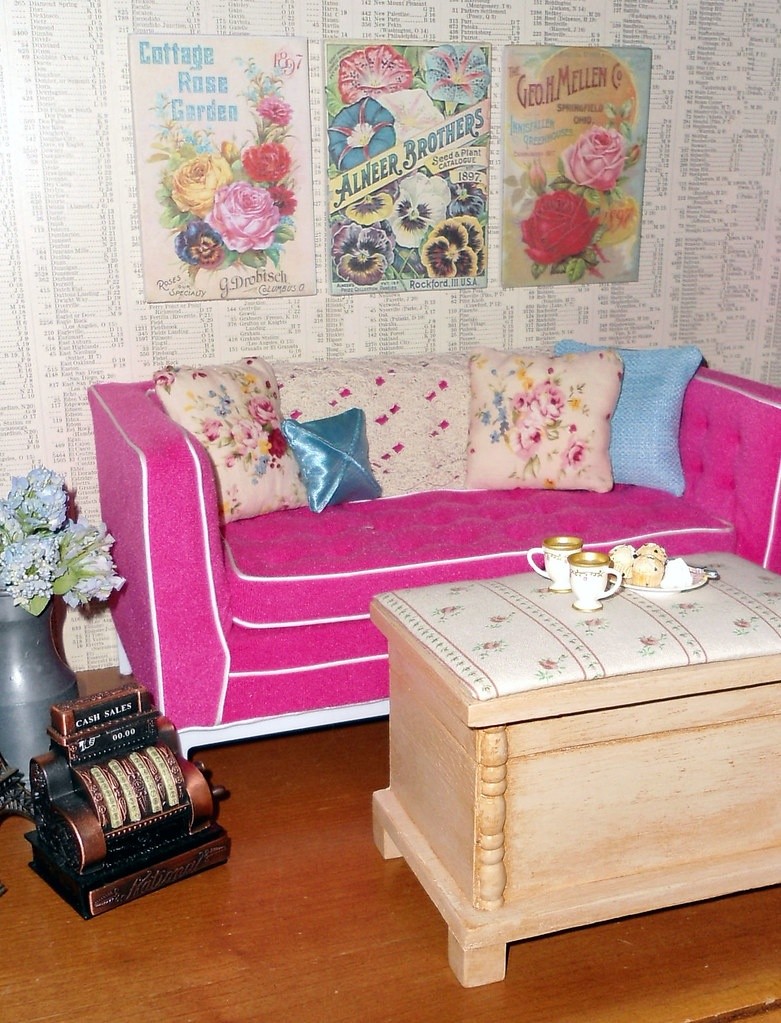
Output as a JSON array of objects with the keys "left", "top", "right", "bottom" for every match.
[{"left": 0, "top": 465, "right": 127, "bottom": 615}]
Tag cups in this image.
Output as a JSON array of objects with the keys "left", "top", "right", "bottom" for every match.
[
  {"left": 526, "top": 536, "right": 584, "bottom": 594},
  {"left": 567, "top": 551, "right": 623, "bottom": 612}
]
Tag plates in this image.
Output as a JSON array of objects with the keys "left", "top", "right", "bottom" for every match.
[{"left": 609, "top": 565, "right": 707, "bottom": 593}]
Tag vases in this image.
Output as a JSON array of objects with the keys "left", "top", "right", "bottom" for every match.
[{"left": 1, "top": 591, "right": 80, "bottom": 793}]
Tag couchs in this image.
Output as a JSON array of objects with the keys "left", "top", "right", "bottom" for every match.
[{"left": 86, "top": 338, "right": 781, "bottom": 758}]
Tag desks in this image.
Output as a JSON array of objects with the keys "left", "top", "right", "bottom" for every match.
[{"left": 367, "top": 551, "right": 781, "bottom": 984}]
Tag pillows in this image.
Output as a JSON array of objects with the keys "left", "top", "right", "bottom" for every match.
[
  {"left": 467, "top": 349, "right": 624, "bottom": 494},
  {"left": 152, "top": 357, "right": 310, "bottom": 525},
  {"left": 554, "top": 341, "right": 702, "bottom": 497},
  {"left": 280, "top": 407, "right": 382, "bottom": 512}
]
